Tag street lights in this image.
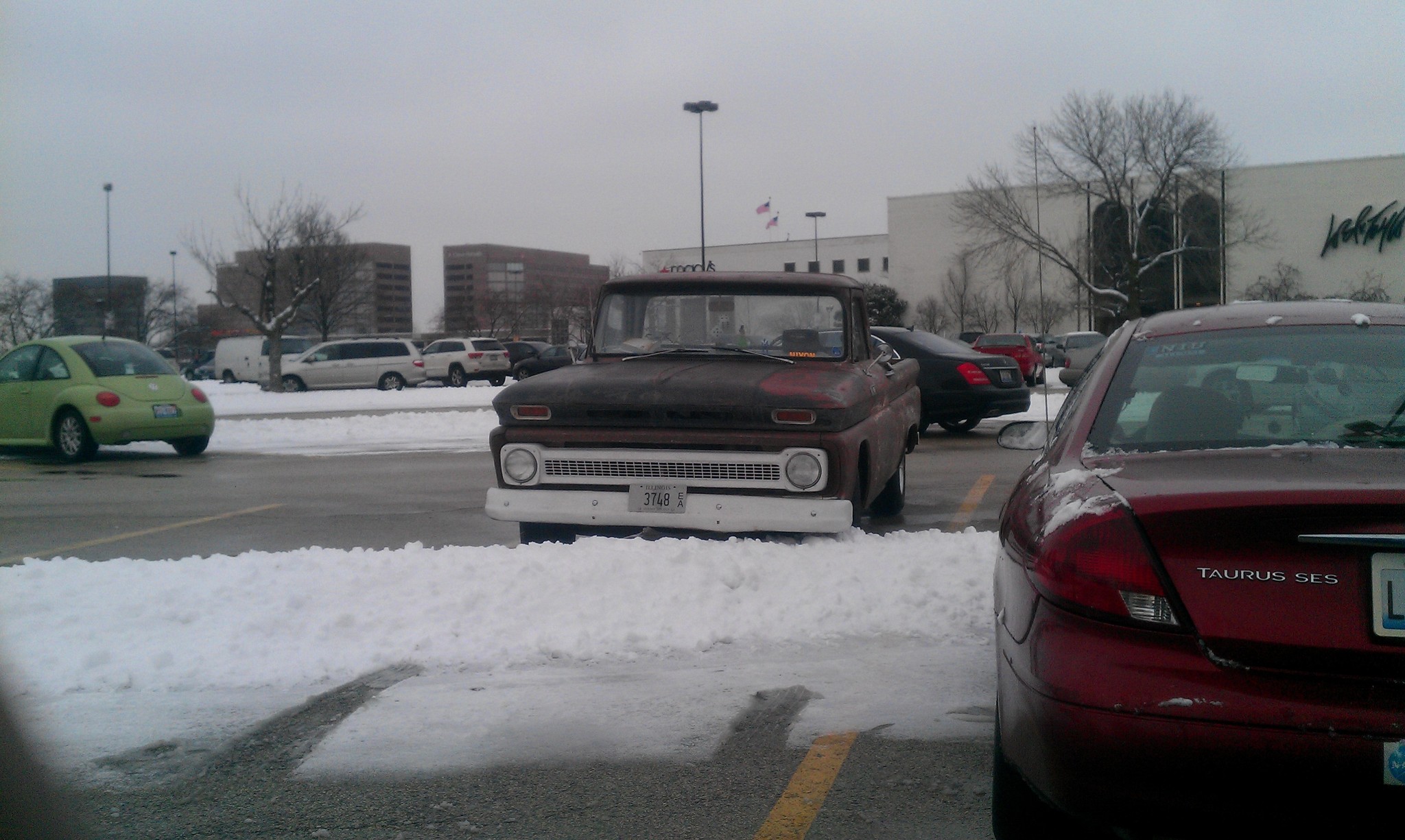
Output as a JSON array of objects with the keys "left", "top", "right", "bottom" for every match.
[
  {"left": 103, "top": 184, "right": 113, "bottom": 336},
  {"left": 683, "top": 101, "right": 719, "bottom": 270},
  {"left": 806, "top": 211, "right": 826, "bottom": 272},
  {"left": 170, "top": 252, "right": 177, "bottom": 359}
]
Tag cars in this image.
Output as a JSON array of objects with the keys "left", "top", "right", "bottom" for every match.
[
  {"left": 1059, "top": 340, "right": 1106, "bottom": 388},
  {"left": 513, "top": 343, "right": 586, "bottom": 382},
  {"left": 181, "top": 350, "right": 214, "bottom": 380},
  {"left": 0, "top": 335, "right": 215, "bottom": 464},
  {"left": 974, "top": 333, "right": 1046, "bottom": 387},
  {"left": 768, "top": 325, "right": 1031, "bottom": 439},
  {"left": 993, "top": 302, "right": 1404, "bottom": 840},
  {"left": 1031, "top": 332, "right": 1106, "bottom": 367},
  {"left": 959, "top": 330, "right": 985, "bottom": 347},
  {"left": 502, "top": 341, "right": 551, "bottom": 376}
]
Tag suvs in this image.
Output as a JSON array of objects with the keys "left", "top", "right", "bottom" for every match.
[{"left": 422, "top": 337, "right": 511, "bottom": 388}]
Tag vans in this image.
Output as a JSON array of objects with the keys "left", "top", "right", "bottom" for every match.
[
  {"left": 282, "top": 335, "right": 426, "bottom": 392},
  {"left": 214, "top": 334, "right": 314, "bottom": 383}
]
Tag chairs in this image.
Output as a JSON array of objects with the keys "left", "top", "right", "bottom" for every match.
[
  {"left": 782, "top": 328, "right": 818, "bottom": 354},
  {"left": 1147, "top": 384, "right": 1236, "bottom": 443}
]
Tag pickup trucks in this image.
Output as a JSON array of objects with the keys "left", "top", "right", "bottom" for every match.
[{"left": 484, "top": 273, "right": 922, "bottom": 545}]
